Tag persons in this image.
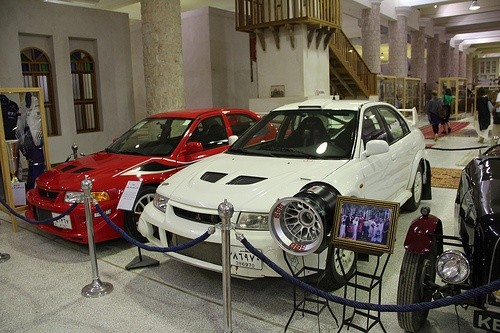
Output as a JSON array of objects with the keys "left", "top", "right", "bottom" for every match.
[
  {"left": 476, "top": 87, "right": 491, "bottom": 143},
  {"left": 469, "top": 92, "right": 475, "bottom": 112},
  {"left": 489, "top": 75, "right": 500, "bottom": 125},
  {"left": 0, "top": 92, "right": 44, "bottom": 206},
  {"left": 338, "top": 210, "right": 389, "bottom": 243},
  {"left": 425, "top": 91, "right": 443, "bottom": 142},
  {"left": 440, "top": 88, "right": 452, "bottom": 134}
]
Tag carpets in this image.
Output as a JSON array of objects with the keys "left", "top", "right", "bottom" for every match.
[{"left": 421, "top": 121, "right": 470, "bottom": 139}]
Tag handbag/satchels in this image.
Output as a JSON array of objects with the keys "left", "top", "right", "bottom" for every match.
[
  {"left": 488, "top": 100, "right": 495, "bottom": 113},
  {"left": 437, "top": 103, "right": 443, "bottom": 118},
  {"left": 440, "top": 106, "right": 446, "bottom": 119}
]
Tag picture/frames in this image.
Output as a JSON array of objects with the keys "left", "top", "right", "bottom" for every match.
[{"left": 330, "top": 195, "right": 401, "bottom": 254}]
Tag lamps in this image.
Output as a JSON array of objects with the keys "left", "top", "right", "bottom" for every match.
[{"left": 468, "top": 0, "right": 481, "bottom": 10}]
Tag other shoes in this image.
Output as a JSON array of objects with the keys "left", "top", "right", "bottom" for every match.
[
  {"left": 441, "top": 131, "right": 446, "bottom": 135},
  {"left": 434, "top": 134, "right": 439, "bottom": 142}
]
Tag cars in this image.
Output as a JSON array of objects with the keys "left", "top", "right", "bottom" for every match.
[
  {"left": 136, "top": 97, "right": 433, "bottom": 291},
  {"left": 396, "top": 125, "right": 500, "bottom": 333},
  {"left": 24, "top": 107, "right": 292, "bottom": 245}
]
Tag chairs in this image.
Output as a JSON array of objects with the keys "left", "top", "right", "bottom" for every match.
[{"left": 284, "top": 116, "right": 332, "bottom": 149}]
